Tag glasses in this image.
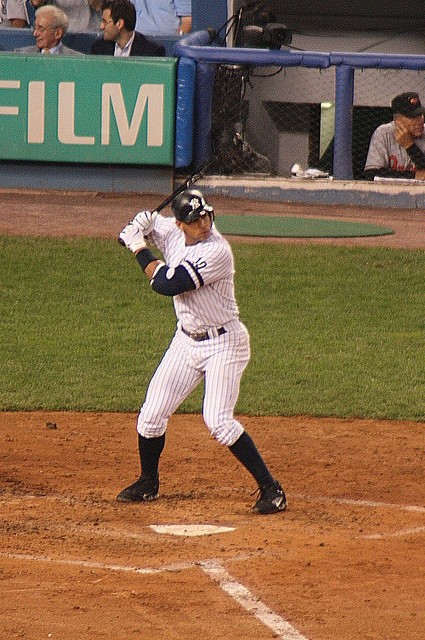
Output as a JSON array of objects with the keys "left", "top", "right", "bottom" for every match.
[{"left": 99, "top": 18, "right": 116, "bottom": 26}]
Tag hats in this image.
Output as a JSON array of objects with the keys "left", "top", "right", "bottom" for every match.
[{"left": 390, "top": 92, "right": 425, "bottom": 118}]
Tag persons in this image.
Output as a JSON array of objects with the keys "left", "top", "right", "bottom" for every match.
[
  {"left": 115, "top": 188, "right": 289, "bottom": 514},
  {"left": 30, "top": 0, "right": 103, "bottom": 33},
  {"left": 90, "top": 0, "right": 166, "bottom": 57},
  {"left": 0, "top": 0, "right": 30, "bottom": 28},
  {"left": 131, "top": 0, "right": 192, "bottom": 37},
  {"left": 12, "top": 5, "right": 89, "bottom": 57},
  {"left": 363, "top": 92, "right": 425, "bottom": 183}
]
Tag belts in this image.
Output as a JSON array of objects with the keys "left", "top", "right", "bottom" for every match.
[{"left": 181, "top": 326, "right": 227, "bottom": 342}]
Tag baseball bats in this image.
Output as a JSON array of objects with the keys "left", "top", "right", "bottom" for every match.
[{"left": 118, "top": 156, "right": 219, "bottom": 246}]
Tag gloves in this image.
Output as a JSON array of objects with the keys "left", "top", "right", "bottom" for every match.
[
  {"left": 119, "top": 224, "right": 146, "bottom": 254},
  {"left": 135, "top": 210, "right": 158, "bottom": 236}
]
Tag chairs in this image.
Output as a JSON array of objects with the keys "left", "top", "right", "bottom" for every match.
[{"left": 0, "top": 28, "right": 187, "bottom": 58}]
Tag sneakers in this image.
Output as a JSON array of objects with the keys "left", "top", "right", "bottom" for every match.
[
  {"left": 251, "top": 480, "right": 287, "bottom": 514},
  {"left": 116, "top": 476, "right": 159, "bottom": 502}
]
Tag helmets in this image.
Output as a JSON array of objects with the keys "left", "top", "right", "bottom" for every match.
[{"left": 171, "top": 190, "right": 214, "bottom": 225}]
